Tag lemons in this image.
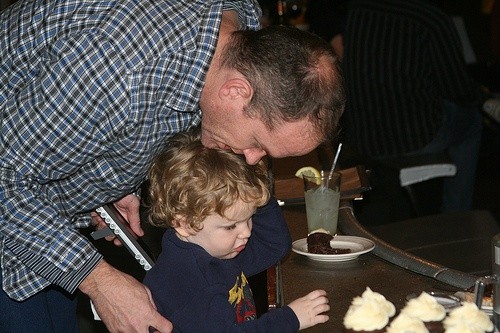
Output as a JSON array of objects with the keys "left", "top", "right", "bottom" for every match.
[{"left": 295, "top": 166, "right": 321, "bottom": 185}]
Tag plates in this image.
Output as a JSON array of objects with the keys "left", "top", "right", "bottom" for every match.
[
  {"left": 405, "top": 293, "right": 460, "bottom": 312},
  {"left": 292, "top": 236, "right": 375, "bottom": 261}
]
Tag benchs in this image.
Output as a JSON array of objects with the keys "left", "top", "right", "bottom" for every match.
[{"left": 360, "top": 211, "right": 499, "bottom": 289}]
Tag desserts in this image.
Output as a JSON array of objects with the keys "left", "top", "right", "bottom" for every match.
[{"left": 343, "top": 285, "right": 494, "bottom": 333}]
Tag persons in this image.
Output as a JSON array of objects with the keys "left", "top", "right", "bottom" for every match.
[
  {"left": 142, "top": 124, "right": 330, "bottom": 333},
  {"left": 0, "top": 0, "right": 348, "bottom": 332},
  {"left": 301, "top": 0, "right": 500, "bottom": 211}
]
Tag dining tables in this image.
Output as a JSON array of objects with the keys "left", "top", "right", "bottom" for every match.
[{"left": 275, "top": 200, "right": 494, "bottom": 333}]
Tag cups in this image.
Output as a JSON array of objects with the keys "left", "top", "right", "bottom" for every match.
[
  {"left": 301, "top": 170, "right": 341, "bottom": 236},
  {"left": 475, "top": 240, "right": 500, "bottom": 332}
]
